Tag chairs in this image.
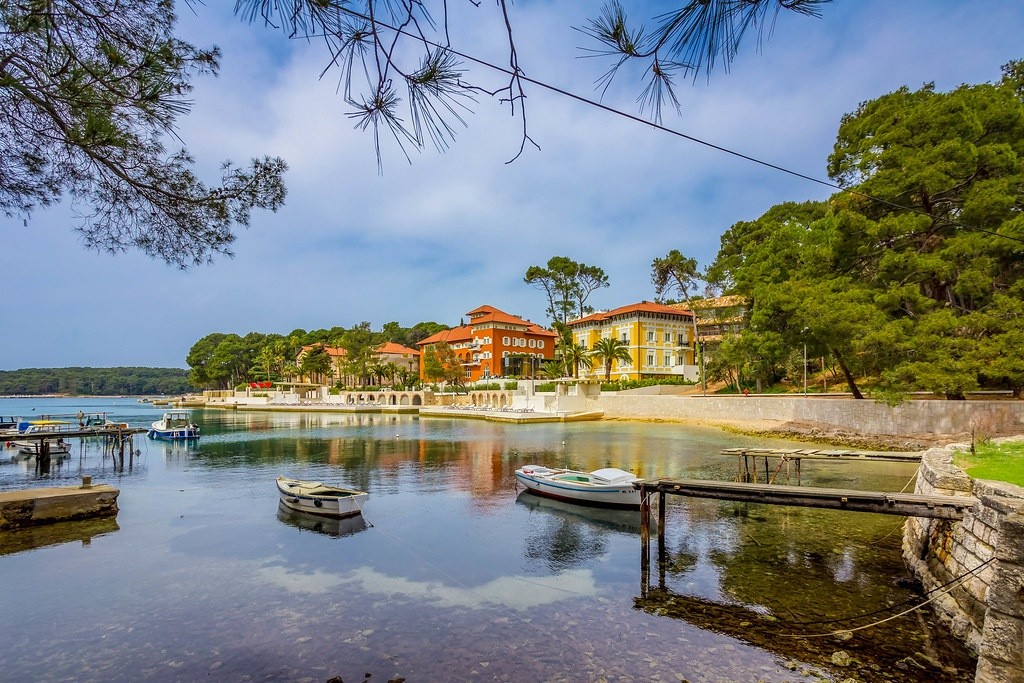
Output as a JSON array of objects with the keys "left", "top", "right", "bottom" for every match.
[{"left": 443, "top": 403, "right": 535, "bottom": 412}]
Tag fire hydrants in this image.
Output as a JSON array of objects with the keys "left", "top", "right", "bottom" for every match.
[{"left": 744, "top": 388, "right": 750, "bottom": 398}]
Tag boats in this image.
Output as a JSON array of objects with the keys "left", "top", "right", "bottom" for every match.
[
  {"left": 7, "top": 440, "right": 73, "bottom": 452},
  {"left": 274, "top": 475, "right": 368, "bottom": 516},
  {"left": 11, "top": 452, "right": 70, "bottom": 464},
  {"left": 275, "top": 500, "right": 372, "bottom": 539},
  {"left": 149, "top": 411, "right": 202, "bottom": 440},
  {"left": 0, "top": 417, "right": 69, "bottom": 438},
  {"left": 76, "top": 412, "right": 130, "bottom": 440},
  {"left": 513, "top": 491, "right": 657, "bottom": 532},
  {"left": 512, "top": 464, "right": 659, "bottom": 509},
  {"left": 137, "top": 397, "right": 151, "bottom": 403}
]
